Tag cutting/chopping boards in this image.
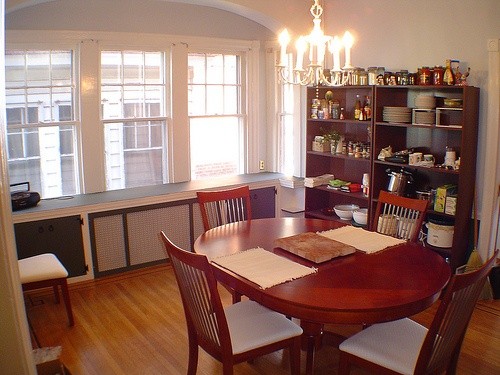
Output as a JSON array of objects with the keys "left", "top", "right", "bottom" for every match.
[{"left": 273, "top": 232, "right": 355, "bottom": 263}]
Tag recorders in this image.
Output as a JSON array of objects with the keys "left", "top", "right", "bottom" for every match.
[{"left": 10, "top": 182, "right": 40, "bottom": 212}]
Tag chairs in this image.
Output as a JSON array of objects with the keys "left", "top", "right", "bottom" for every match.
[
  {"left": 160, "top": 186, "right": 500, "bottom": 375},
  {"left": 18, "top": 252, "right": 74, "bottom": 326}
]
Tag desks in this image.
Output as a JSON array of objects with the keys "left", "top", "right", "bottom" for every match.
[{"left": 193, "top": 217, "right": 453, "bottom": 375}]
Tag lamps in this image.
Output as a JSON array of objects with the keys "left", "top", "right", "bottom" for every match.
[{"left": 276, "top": 0, "right": 358, "bottom": 87}]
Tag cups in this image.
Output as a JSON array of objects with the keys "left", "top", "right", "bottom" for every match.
[
  {"left": 424, "top": 154, "right": 436, "bottom": 164},
  {"left": 414, "top": 152, "right": 423, "bottom": 160},
  {"left": 408, "top": 154, "right": 418, "bottom": 166}
]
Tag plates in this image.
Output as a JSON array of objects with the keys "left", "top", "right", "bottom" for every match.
[
  {"left": 376, "top": 214, "right": 399, "bottom": 236},
  {"left": 381, "top": 96, "right": 437, "bottom": 124}
]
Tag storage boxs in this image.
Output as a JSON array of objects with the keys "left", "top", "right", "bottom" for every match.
[{"left": 433, "top": 183, "right": 458, "bottom": 215}]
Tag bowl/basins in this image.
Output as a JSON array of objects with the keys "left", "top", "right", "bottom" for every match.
[
  {"left": 352, "top": 209, "right": 368, "bottom": 224},
  {"left": 333, "top": 205, "right": 361, "bottom": 221},
  {"left": 444, "top": 100, "right": 462, "bottom": 106}
]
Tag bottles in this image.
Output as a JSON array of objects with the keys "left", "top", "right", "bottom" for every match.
[
  {"left": 340, "top": 108, "right": 345, "bottom": 119},
  {"left": 354, "top": 93, "right": 370, "bottom": 121},
  {"left": 397, "top": 59, "right": 459, "bottom": 86}
]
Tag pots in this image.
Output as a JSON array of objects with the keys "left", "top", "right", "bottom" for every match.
[
  {"left": 427, "top": 218, "right": 454, "bottom": 248},
  {"left": 397, "top": 211, "right": 426, "bottom": 240},
  {"left": 387, "top": 167, "right": 415, "bottom": 193}
]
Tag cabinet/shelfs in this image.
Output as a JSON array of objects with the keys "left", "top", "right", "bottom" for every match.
[
  {"left": 370, "top": 85, "right": 480, "bottom": 263},
  {"left": 304, "top": 86, "right": 375, "bottom": 230},
  {"left": 13, "top": 215, "right": 89, "bottom": 278},
  {"left": 226, "top": 185, "right": 279, "bottom": 224}
]
